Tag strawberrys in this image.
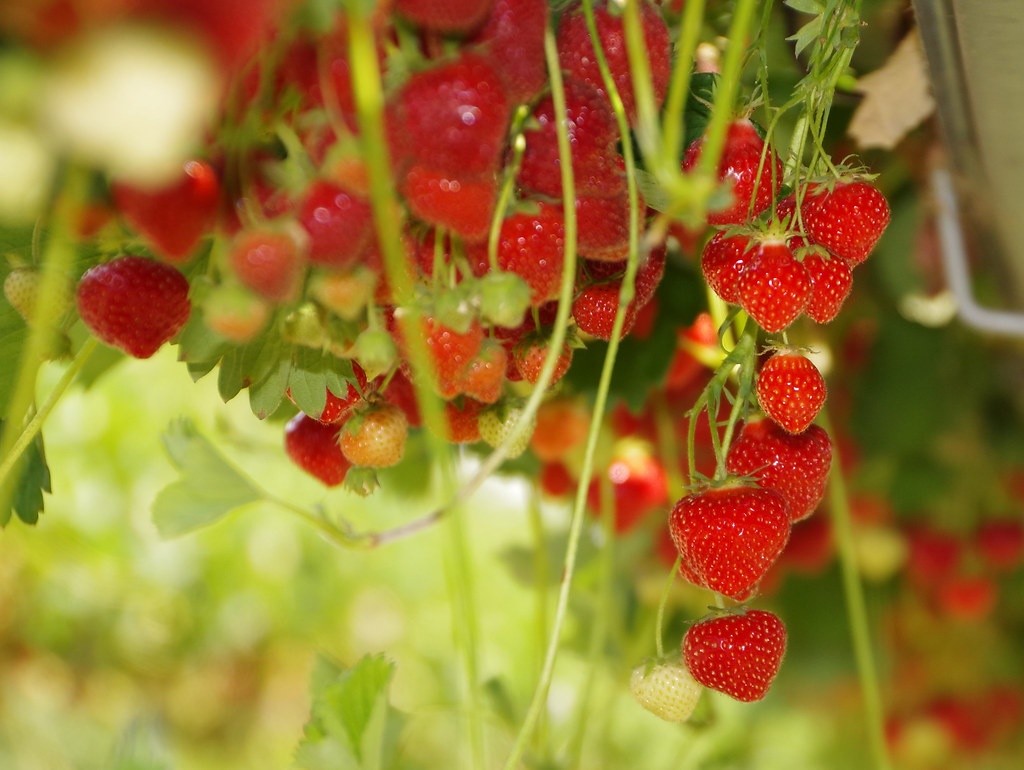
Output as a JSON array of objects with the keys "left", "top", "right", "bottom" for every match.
[{"left": 3, "top": 0, "right": 892, "bottom": 725}]
